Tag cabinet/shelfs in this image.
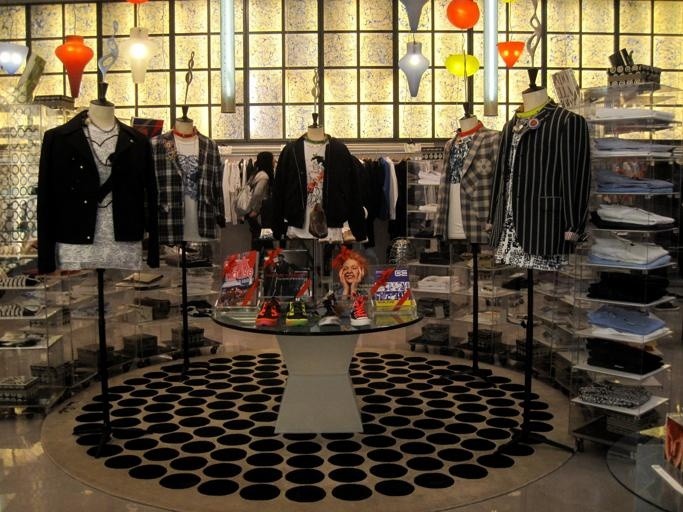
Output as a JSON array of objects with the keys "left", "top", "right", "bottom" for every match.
[
  {"left": 405, "top": 82, "right": 682, "bottom": 465},
  {"left": 0, "top": 106, "right": 221, "bottom": 421}
]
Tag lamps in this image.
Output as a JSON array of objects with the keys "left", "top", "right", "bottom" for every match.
[
  {"left": 397, "top": 1, "right": 524, "bottom": 115},
  {"left": 221, "top": 0, "right": 235, "bottom": 112},
  {"left": 2, "top": 0, "right": 148, "bottom": 100}
]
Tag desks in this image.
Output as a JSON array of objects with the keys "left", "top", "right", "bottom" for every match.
[
  {"left": 607, "top": 427, "right": 683, "bottom": 512},
  {"left": 212, "top": 305, "right": 423, "bottom": 434}
]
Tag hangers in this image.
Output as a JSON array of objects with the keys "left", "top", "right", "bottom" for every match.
[
  {"left": 222, "top": 152, "right": 281, "bottom": 167},
  {"left": 353, "top": 150, "right": 416, "bottom": 168}
]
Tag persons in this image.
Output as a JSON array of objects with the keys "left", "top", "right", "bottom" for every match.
[
  {"left": 481, "top": 84, "right": 593, "bottom": 275},
  {"left": 216, "top": 250, "right": 314, "bottom": 309},
  {"left": 256, "top": 125, "right": 374, "bottom": 246},
  {"left": 330, "top": 248, "right": 370, "bottom": 300},
  {"left": 147, "top": 117, "right": 230, "bottom": 246},
  {"left": 434, "top": 113, "right": 505, "bottom": 247},
  {"left": 239, "top": 150, "right": 276, "bottom": 264},
  {"left": 32, "top": 94, "right": 164, "bottom": 278}
]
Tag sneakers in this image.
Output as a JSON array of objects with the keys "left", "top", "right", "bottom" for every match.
[
  {"left": 317, "top": 289, "right": 341, "bottom": 326},
  {"left": 285, "top": 296, "right": 308, "bottom": 327},
  {"left": 255, "top": 296, "right": 282, "bottom": 327},
  {"left": 350, "top": 289, "right": 372, "bottom": 327}
]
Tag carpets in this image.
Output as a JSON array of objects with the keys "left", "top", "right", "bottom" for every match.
[{"left": 41, "top": 348, "right": 584, "bottom": 512}]
